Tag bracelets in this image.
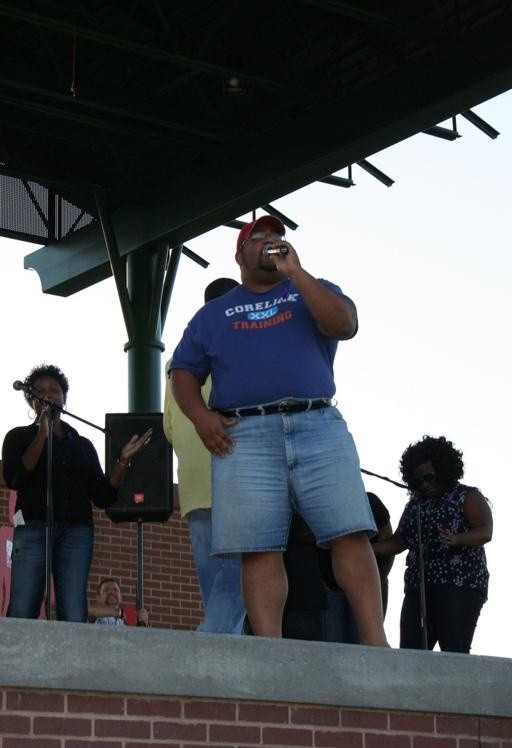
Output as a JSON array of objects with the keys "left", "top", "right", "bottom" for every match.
[{"left": 116, "top": 458, "right": 132, "bottom": 468}]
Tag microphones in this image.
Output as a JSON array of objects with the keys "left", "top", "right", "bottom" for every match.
[
  {"left": 13, "top": 380, "right": 32, "bottom": 392},
  {"left": 261, "top": 243, "right": 288, "bottom": 256}
]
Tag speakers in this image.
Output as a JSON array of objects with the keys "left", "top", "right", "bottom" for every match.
[{"left": 104, "top": 412, "right": 174, "bottom": 524}]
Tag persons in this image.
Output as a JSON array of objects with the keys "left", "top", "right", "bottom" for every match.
[
  {"left": 2, "top": 364, "right": 155, "bottom": 623},
  {"left": 370, "top": 434, "right": 492, "bottom": 654},
  {"left": 86, "top": 579, "right": 149, "bottom": 627},
  {"left": 163, "top": 279, "right": 249, "bottom": 637},
  {"left": 362, "top": 492, "right": 393, "bottom": 619},
  {"left": 168, "top": 215, "right": 389, "bottom": 647}
]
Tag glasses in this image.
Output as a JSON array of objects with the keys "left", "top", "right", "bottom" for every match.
[
  {"left": 414, "top": 472, "right": 437, "bottom": 484},
  {"left": 240, "top": 229, "right": 283, "bottom": 249}
]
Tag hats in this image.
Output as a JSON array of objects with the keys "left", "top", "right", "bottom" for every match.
[{"left": 236, "top": 215, "right": 286, "bottom": 251}]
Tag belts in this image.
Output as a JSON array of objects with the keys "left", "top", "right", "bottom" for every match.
[{"left": 221, "top": 399, "right": 332, "bottom": 418}]
